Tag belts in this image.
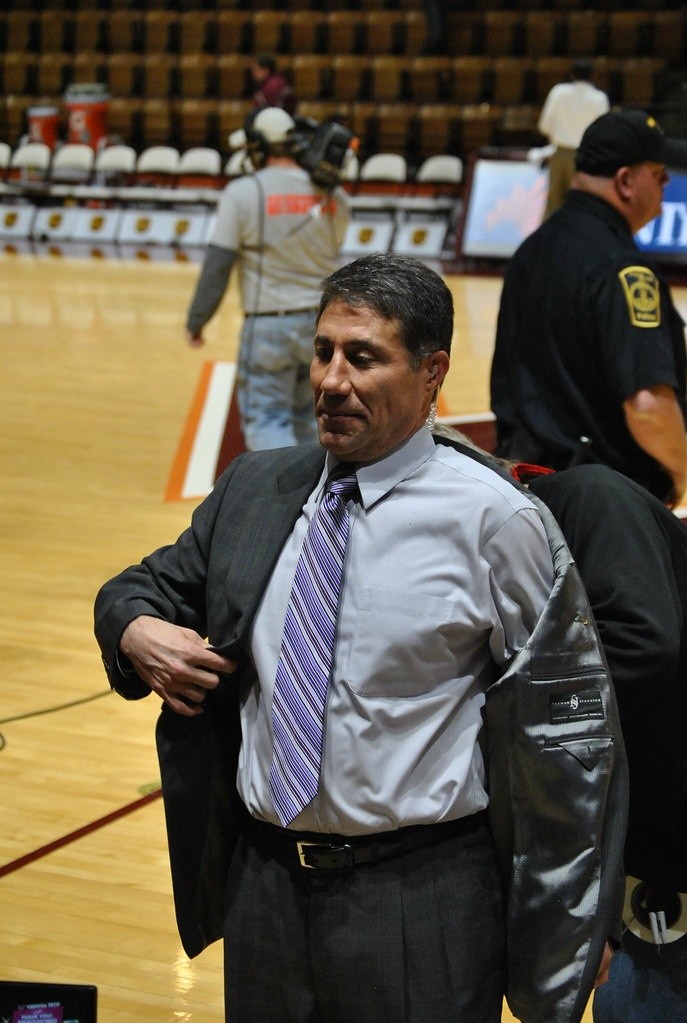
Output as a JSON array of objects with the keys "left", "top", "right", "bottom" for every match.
[
  {"left": 245, "top": 308, "right": 321, "bottom": 318},
  {"left": 260, "top": 812, "right": 487, "bottom": 868}
]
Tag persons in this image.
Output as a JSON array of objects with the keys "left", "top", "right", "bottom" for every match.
[
  {"left": 534, "top": 62, "right": 613, "bottom": 219},
  {"left": 92, "top": 249, "right": 632, "bottom": 1023},
  {"left": 184, "top": 51, "right": 363, "bottom": 453},
  {"left": 487, "top": 107, "right": 687, "bottom": 528}
]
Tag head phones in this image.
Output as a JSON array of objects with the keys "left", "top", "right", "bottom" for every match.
[{"left": 244, "top": 104, "right": 270, "bottom": 169}]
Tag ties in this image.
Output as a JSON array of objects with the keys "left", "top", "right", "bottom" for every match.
[{"left": 270, "top": 469, "right": 358, "bottom": 828}]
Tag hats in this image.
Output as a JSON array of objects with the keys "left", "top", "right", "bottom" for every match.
[
  {"left": 249, "top": 106, "right": 294, "bottom": 145},
  {"left": 577, "top": 107, "right": 686, "bottom": 161}
]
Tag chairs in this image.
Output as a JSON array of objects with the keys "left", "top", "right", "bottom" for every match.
[{"left": 0, "top": 143, "right": 463, "bottom": 221}]
